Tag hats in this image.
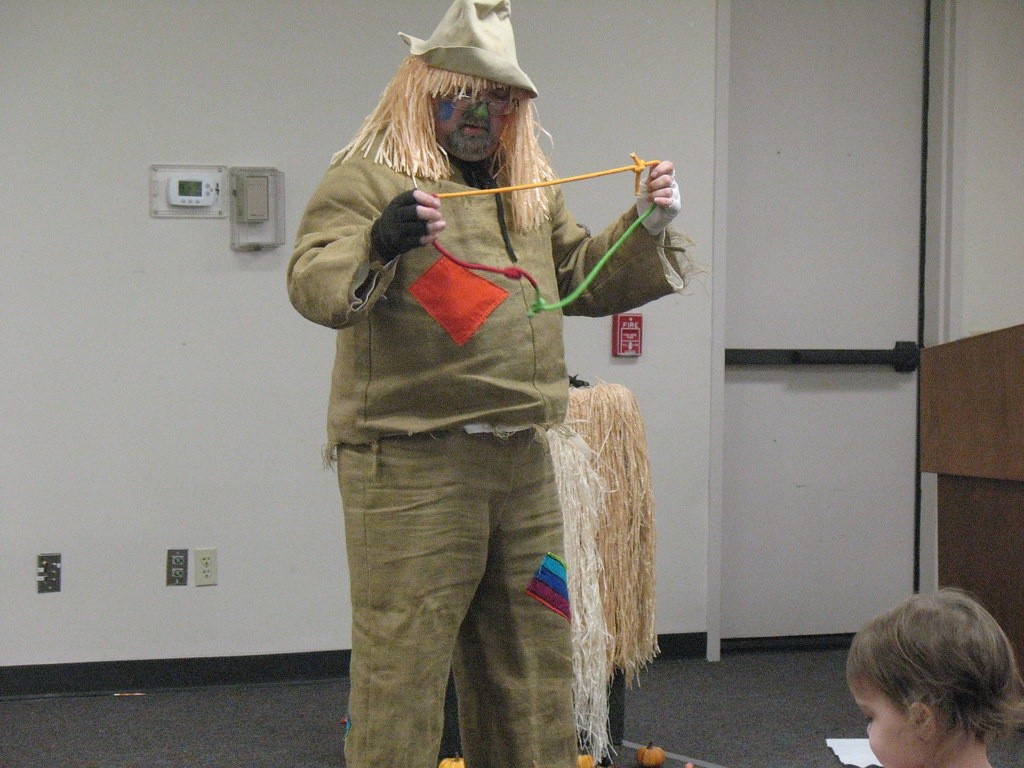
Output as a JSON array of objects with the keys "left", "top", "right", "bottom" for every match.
[{"left": 398, "top": 0, "right": 539, "bottom": 100}]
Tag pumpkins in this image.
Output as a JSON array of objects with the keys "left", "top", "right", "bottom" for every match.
[
  {"left": 636, "top": 740, "right": 665, "bottom": 768},
  {"left": 576, "top": 750, "right": 594, "bottom": 768},
  {"left": 438, "top": 751, "right": 465, "bottom": 768}
]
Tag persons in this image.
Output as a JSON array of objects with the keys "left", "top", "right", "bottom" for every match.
[
  {"left": 844, "top": 590, "right": 1024, "bottom": 768},
  {"left": 287, "top": 0, "right": 685, "bottom": 768}
]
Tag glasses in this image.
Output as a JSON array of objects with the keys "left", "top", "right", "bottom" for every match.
[{"left": 444, "top": 91, "right": 518, "bottom": 115}]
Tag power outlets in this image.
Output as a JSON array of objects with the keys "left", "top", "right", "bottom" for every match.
[{"left": 194, "top": 547, "right": 219, "bottom": 587}]
